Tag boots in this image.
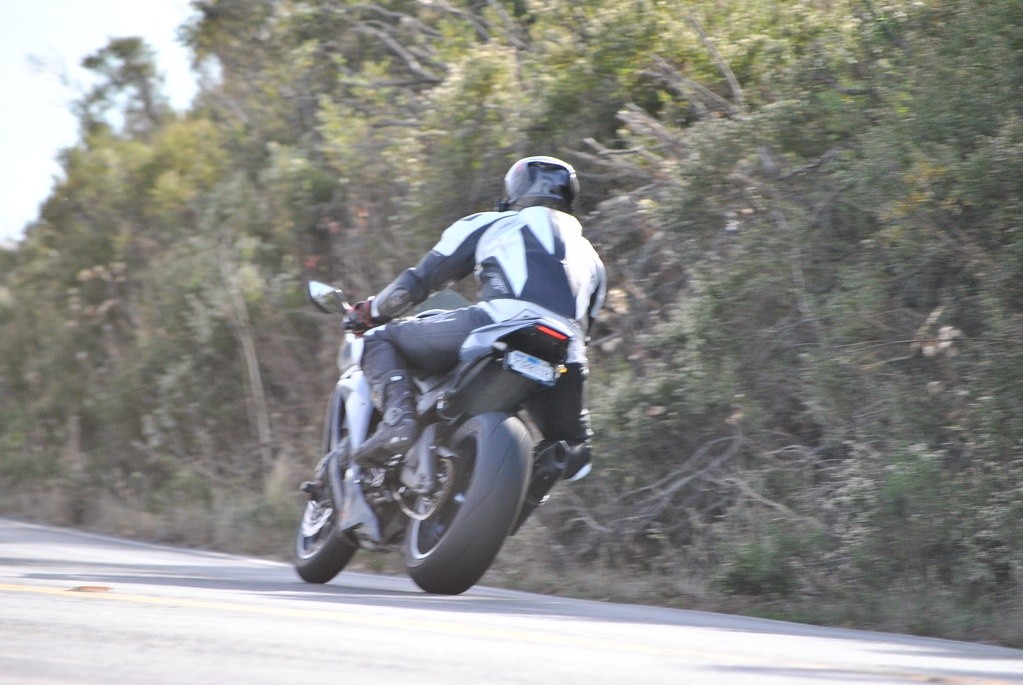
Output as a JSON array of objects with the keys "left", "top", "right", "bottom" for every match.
[{"left": 349, "top": 369, "right": 419, "bottom": 466}]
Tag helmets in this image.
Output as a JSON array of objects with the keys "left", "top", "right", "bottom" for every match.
[{"left": 502, "top": 154, "right": 580, "bottom": 215}]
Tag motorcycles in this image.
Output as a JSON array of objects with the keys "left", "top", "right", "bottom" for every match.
[{"left": 288, "top": 276, "right": 595, "bottom": 595}]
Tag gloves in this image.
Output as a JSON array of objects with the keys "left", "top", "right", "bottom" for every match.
[{"left": 345, "top": 300, "right": 381, "bottom": 336}]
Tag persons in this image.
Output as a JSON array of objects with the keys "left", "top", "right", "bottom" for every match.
[{"left": 342, "top": 156, "right": 607, "bottom": 534}]
